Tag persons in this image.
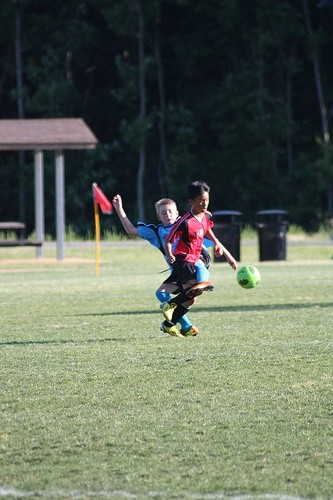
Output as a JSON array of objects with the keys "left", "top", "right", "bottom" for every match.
[
  {"left": 113, "top": 195, "right": 238, "bottom": 336},
  {"left": 159, "top": 181, "right": 225, "bottom": 338}
]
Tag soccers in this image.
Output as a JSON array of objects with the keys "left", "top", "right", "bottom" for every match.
[{"left": 237, "top": 264, "right": 261, "bottom": 290}]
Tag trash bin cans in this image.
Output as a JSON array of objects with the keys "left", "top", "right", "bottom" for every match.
[
  {"left": 211, "top": 211, "right": 244, "bottom": 263},
  {"left": 254, "top": 210, "right": 289, "bottom": 262}
]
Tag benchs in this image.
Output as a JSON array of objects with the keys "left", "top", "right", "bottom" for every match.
[{"left": 0, "top": 222, "right": 45, "bottom": 258}]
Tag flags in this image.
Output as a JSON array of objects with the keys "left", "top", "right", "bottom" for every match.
[{"left": 93, "top": 183, "right": 112, "bottom": 214}]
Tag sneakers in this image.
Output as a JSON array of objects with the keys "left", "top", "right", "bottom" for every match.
[
  {"left": 186, "top": 280, "right": 215, "bottom": 298},
  {"left": 159, "top": 320, "right": 182, "bottom": 337},
  {"left": 160, "top": 302, "right": 177, "bottom": 323},
  {"left": 179, "top": 325, "right": 199, "bottom": 336}
]
